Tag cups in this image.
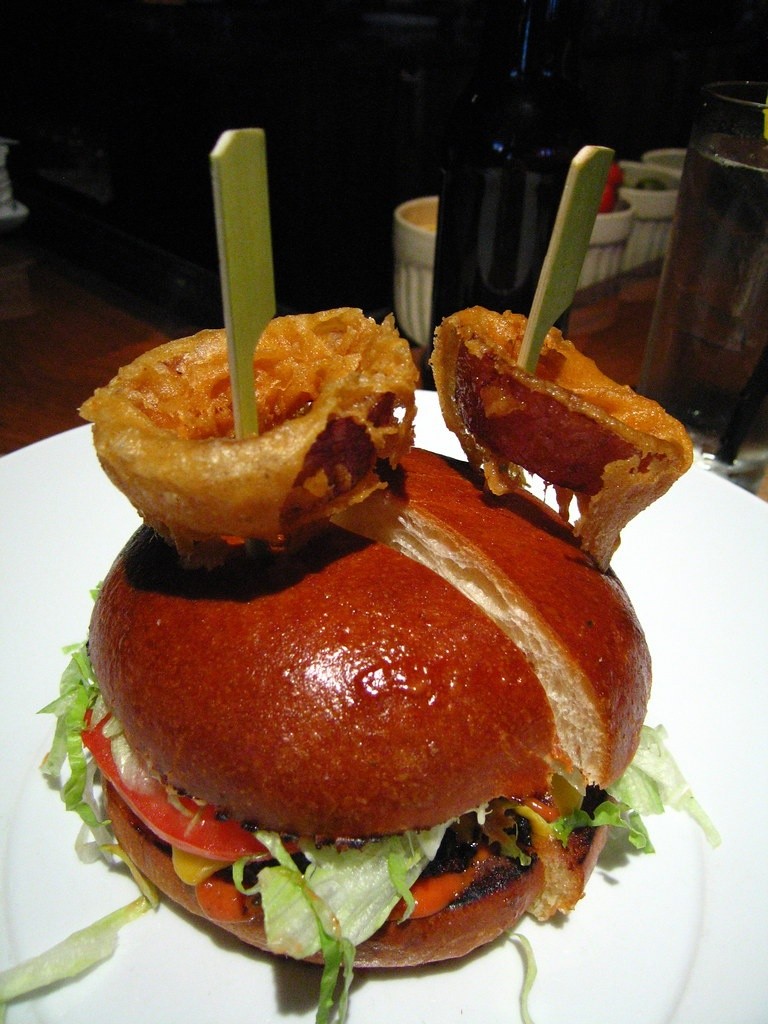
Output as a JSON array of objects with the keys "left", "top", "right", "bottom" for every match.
[
  {"left": 642, "top": 149, "right": 688, "bottom": 174},
  {"left": 638, "top": 81, "right": 768, "bottom": 435},
  {"left": 393, "top": 196, "right": 438, "bottom": 349},
  {"left": 613, "top": 160, "right": 679, "bottom": 270},
  {"left": 578, "top": 204, "right": 628, "bottom": 297}
]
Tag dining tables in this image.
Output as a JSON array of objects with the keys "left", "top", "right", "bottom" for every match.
[{"left": 1, "top": 387, "right": 768, "bottom": 1023}]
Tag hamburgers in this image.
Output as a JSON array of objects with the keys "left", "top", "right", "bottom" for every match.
[{"left": 0, "top": 448, "right": 720, "bottom": 1024}]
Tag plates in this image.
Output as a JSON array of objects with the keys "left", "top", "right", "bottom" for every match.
[{"left": 3, "top": 386, "right": 768, "bottom": 1020}]
